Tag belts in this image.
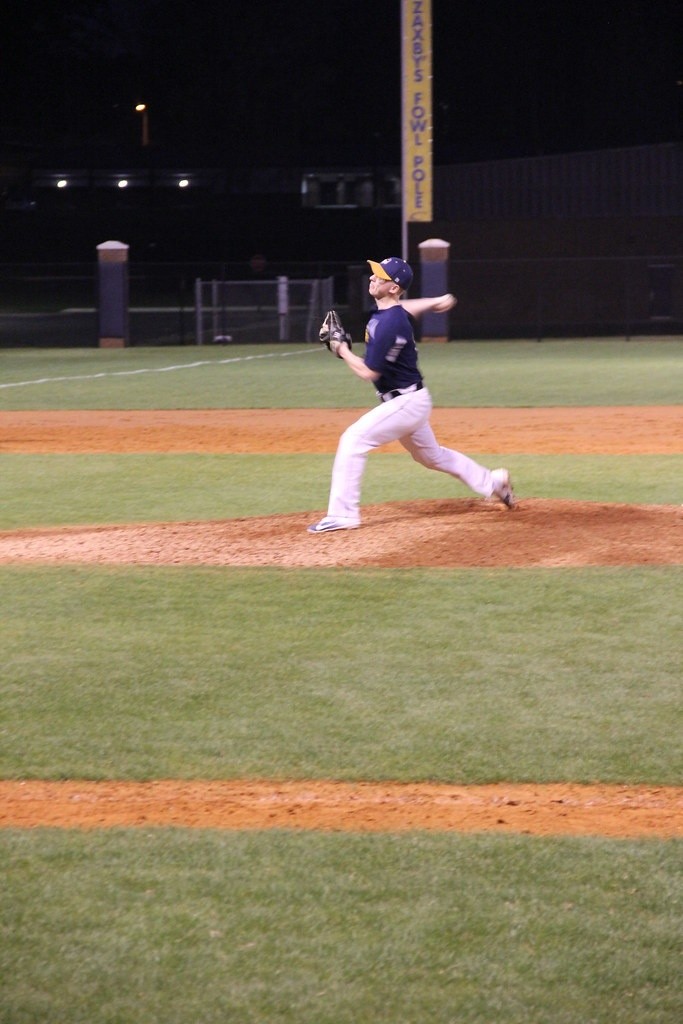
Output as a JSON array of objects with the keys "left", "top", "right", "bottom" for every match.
[{"left": 378, "top": 382, "right": 423, "bottom": 401}]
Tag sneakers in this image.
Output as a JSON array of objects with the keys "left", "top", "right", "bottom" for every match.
[
  {"left": 492, "top": 468, "right": 515, "bottom": 509},
  {"left": 306, "top": 516, "right": 360, "bottom": 534}
]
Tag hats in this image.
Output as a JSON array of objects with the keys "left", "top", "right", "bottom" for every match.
[{"left": 366, "top": 258, "right": 414, "bottom": 291}]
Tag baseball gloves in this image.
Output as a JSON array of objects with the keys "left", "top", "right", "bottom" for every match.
[{"left": 317, "top": 310, "right": 352, "bottom": 358}]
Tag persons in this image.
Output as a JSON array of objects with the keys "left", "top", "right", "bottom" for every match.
[{"left": 307, "top": 257, "right": 512, "bottom": 534}]
